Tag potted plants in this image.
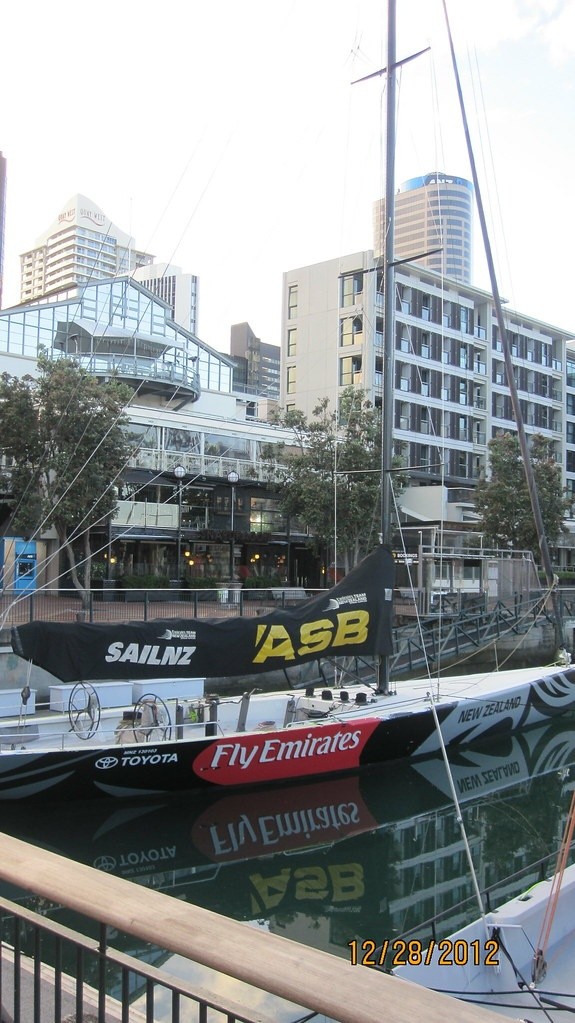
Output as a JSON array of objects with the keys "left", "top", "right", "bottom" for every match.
[
  {"left": 183, "top": 577, "right": 229, "bottom": 600},
  {"left": 114, "top": 574, "right": 174, "bottom": 603},
  {"left": 243, "top": 577, "right": 281, "bottom": 600}
]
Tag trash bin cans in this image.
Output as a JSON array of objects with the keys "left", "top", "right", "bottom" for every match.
[
  {"left": 215, "top": 583, "right": 230, "bottom": 604},
  {"left": 229, "top": 583, "right": 243, "bottom": 603},
  {"left": 103, "top": 580, "right": 115, "bottom": 602},
  {"left": 530, "top": 588, "right": 541, "bottom": 615}
]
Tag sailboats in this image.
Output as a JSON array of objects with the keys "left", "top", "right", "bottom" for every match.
[
  {"left": 0, "top": 0, "right": 575, "bottom": 824},
  {"left": 0, "top": 696, "right": 575, "bottom": 1020}
]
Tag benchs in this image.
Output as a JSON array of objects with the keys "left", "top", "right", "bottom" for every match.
[
  {"left": 268, "top": 586, "right": 312, "bottom": 604},
  {"left": 128, "top": 678, "right": 206, "bottom": 704},
  {"left": 399, "top": 586, "right": 427, "bottom": 605},
  {"left": 0, "top": 688, "right": 38, "bottom": 717},
  {"left": 48, "top": 681, "right": 133, "bottom": 711}
]
